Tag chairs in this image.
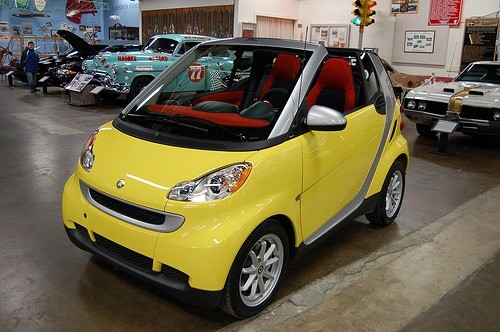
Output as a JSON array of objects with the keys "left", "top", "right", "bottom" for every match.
[
  {"left": 257, "top": 54, "right": 302, "bottom": 111},
  {"left": 306, "top": 58, "right": 356, "bottom": 113}
]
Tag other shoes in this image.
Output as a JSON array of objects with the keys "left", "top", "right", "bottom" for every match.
[
  {"left": 31, "top": 91, "right": 36, "bottom": 93},
  {"left": 36, "top": 89, "right": 40, "bottom": 91}
]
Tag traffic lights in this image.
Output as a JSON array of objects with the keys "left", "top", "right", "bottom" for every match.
[{"left": 349, "top": 0, "right": 377, "bottom": 27}]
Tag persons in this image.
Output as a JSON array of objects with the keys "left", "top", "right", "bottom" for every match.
[{"left": 20, "top": 41, "right": 40, "bottom": 92}]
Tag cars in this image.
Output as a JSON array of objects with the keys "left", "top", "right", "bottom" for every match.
[
  {"left": 13, "top": 46, "right": 80, "bottom": 85},
  {"left": 48, "top": 30, "right": 108, "bottom": 84},
  {"left": 402, "top": 60, "right": 500, "bottom": 138},
  {"left": 82, "top": 34, "right": 250, "bottom": 106},
  {"left": 61, "top": 37, "right": 410, "bottom": 320}
]
